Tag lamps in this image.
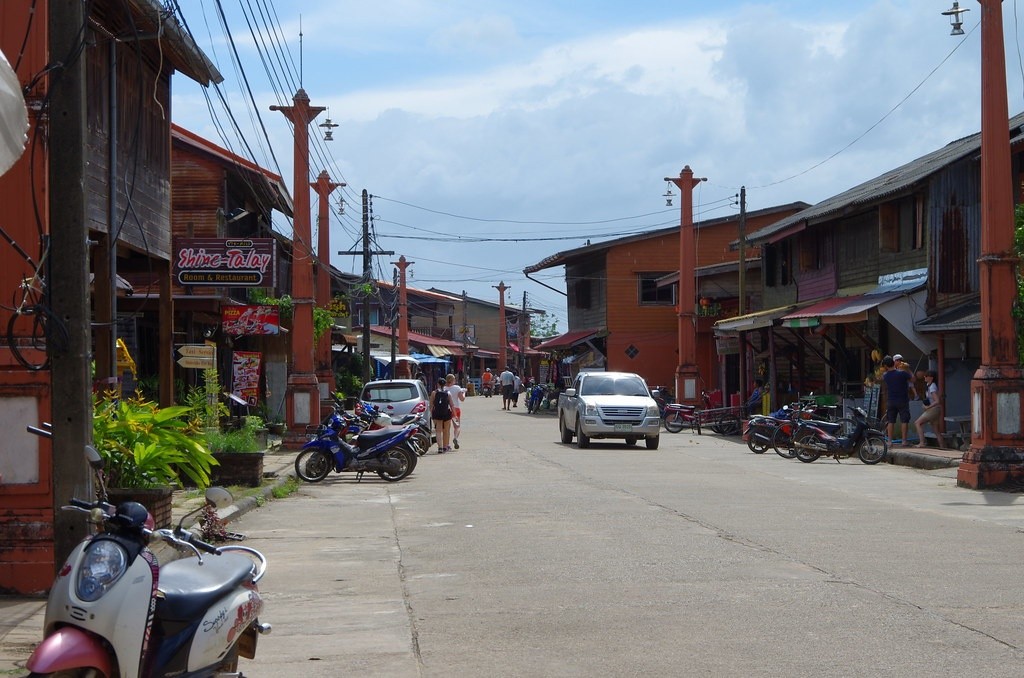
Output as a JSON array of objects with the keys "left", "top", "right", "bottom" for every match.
[
  {"left": 225, "top": 207, "right": 249, "bottom": 222},
  {"left": 25, "top": 89, "right": 49, "bottom": 113}
]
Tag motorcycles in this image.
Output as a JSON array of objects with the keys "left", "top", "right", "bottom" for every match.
[{"left": 293, "top": 391, "right": 434, "bottom": 483}]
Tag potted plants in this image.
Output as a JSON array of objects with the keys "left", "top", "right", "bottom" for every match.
[{"left": 95, "top": 382, "right": 286, "bottom": 532}]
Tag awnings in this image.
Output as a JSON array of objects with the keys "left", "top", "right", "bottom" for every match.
[
  {"left": 780, "top": 282, "right": 926, "bottom": 376},
  {"left": 372, "top": 352, "right": 453, "bottom": 366},
  {"left": 533, "top": 327, "right": 607, "bottom": 359},
  {"left": 714, "top": 296, "right": 830, "bottom": 372}
]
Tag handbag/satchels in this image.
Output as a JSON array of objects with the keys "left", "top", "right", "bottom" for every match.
[{"left": 520, "top": 385, "right": 526, "bottom": 393}]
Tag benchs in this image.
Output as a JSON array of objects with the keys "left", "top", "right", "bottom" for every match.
[{"left": 925, "top": 431, "right": 971, "bottom": 449}]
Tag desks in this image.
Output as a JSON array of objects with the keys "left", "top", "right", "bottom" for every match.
[{"left": 942, "top": 415, "right": 971, "bottom": 444}]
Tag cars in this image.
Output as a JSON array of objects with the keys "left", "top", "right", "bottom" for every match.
[{"left": 359, "top": 379, "right": 437, "bottom": 447}]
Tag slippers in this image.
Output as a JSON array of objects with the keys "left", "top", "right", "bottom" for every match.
[
  {"left": 915, "top": 445, "right": 926, "bottom": 448},
  {"left": 886, "top": 444, "right": 892, "bottom": 449},
  {"left": 902, "top": 444, "right": 914, "bottom": 448}
]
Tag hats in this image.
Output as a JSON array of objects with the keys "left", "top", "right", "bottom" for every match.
[
  {"left": 485, "top": 368, "right": 490, "bottom": 372},
  {"left": 504, "top": 366, "right": 509, "bottom": 369},
  {"left": 892, "top": 354, "right": 902, "bottom": 361}
]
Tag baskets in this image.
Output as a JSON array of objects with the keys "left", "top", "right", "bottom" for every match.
[{"left": 305, "top": 424, "right": 324, "bottom": 438}]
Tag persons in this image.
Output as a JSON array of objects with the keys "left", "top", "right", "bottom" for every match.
[
  {"left": 444, "top": 374, "right": 465, "bottom": 452},
  {"left": 747, "top": 379, "right": 763, "bottom": 404},
  {"left": 512, "top": 371, "right": 522, "bottom": 407},
  {"left": 415, "top": 367, "right": 427, "bottom": 389},
  {"left": 500, "top": 366, "right": 515, "bottom": 411},
  {"left": 480, "top": 367, "right": 494, "bottom": 396},
  {"left": 882, "top": 354, "right": 920, "bottom": 447},
  {"left": 912, "top": 370, "right": 943, "bottom": 448},
  {"left": 430, "top": 378, "right": 456, "bottom": 453}
]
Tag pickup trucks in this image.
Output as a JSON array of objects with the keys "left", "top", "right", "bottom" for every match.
[{"left": 557, "top": 371, "right": 661, "bottom": 450}]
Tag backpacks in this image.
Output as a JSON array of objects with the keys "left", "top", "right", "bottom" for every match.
[{"left": 432, "top": 390, "right": 451, "bottom": 420}]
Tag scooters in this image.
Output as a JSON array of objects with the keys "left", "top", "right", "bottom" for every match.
[
  {"left": 22, "top": 444, "right": 275, "bottom": 678},
  {"left": 649, "top": 385, "right": 888, "bottom": 466},
  {"left": 523, "top": 382, "right": 553, "bottom": 415}
]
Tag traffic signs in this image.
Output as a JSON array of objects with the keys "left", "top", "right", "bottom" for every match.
[
  {"left": 176, "top": 356, "right": 213, "bottom": 369},
  {"left": 177, "top": 345, "right": 215, "bottom": 358}
]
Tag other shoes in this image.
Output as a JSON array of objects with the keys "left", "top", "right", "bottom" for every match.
[
  {"left": 448, "top": 447, "right": 452, "bottom": 451},
  {"left": 914, "top": 396, "right": 919, "bottom": 400},
  {"left": 502, "top": 408, "right": 506, "bottom": 410},
  {"left": 438, "top": 448, "right": 443, "bottom": 453},
  {"left": 507, "top": 409, "right": 511, "bottom": 410},
  {"left": 513, "top": 406, "right": 517, "bottom": 407},
  {"left": 453, "top": 438, "right": 460, "bottom": 449},
  {"left": 443, "top": 448, "right": 447, "bottom": 452}
]
